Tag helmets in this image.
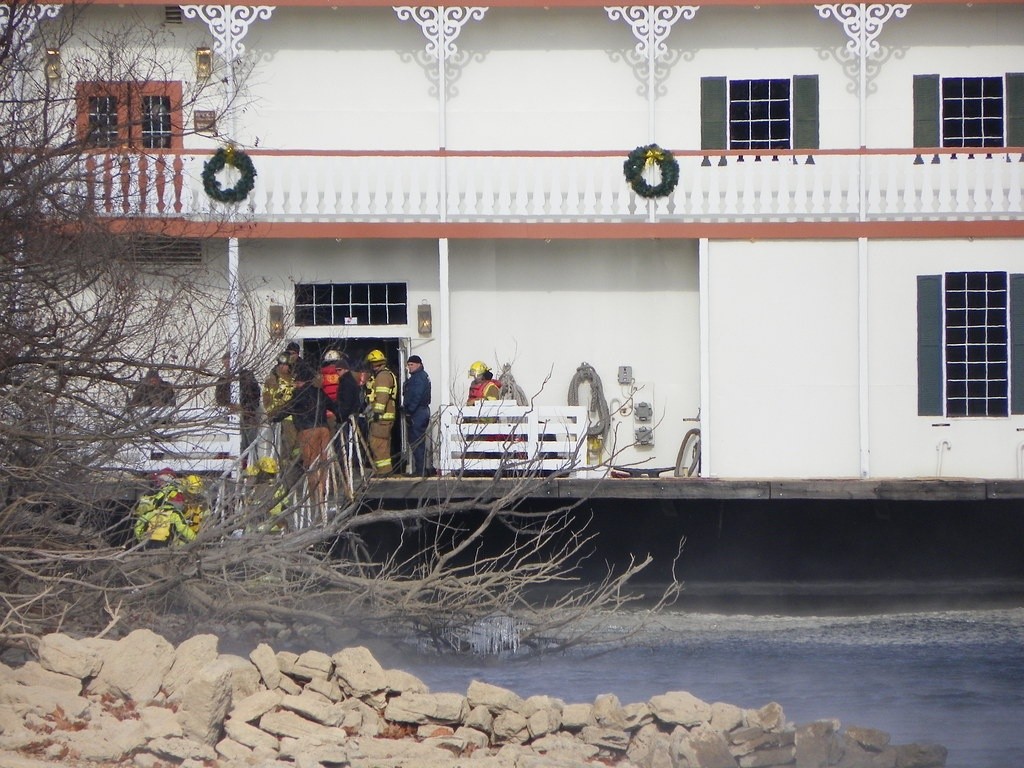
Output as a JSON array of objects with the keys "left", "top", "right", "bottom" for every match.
[
  {"left": 368, "top": 349, "right": 386, "bottom": 362},
  {"left": 325, "top": 350, "right": 342, "bottom": 361},
  {"left": 470, "top": 361, "right": 488, "bottom": 376},
  {"left": 183, "top": 475, "right": 203, "bottom": 493},
  {"left": 155, "top": 468, "right": 178, "bottom": 484},
  {"left": 278, "top": 352, "right": 294, "bottom": 365},
  {"left": 254, "top": 457, "right": 280, "bottom": 474}
]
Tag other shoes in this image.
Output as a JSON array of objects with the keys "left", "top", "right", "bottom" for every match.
[
  {"left": 427, "top": 469, "right": 437, "bottom": 476},
  {"left": 409, "top": 469, "right": 427, "bottom": 477},
  {"left": 369, "top": 473, "right": 388, "bottom": 478}
]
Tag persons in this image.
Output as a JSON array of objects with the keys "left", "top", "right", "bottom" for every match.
[
  {"left": 455, "top": 362, "right": 499, "bottom": 477},
  {"left": 244, "top": 458, "right": 289, "bottom": 588},
  {"left": 403, "top": 355, "right": 431, "bottom": 477},
  {"left": 263, "top": 342, "right": 361, "bottom": 501},
  {"left": 365, "top": 350, "right": 397, "bottom": 477},
  {"left": 216, "top": 352, "right": 260, "bottom": 474},
  {"left": 135, "top": 370, "right": 175, "bottom": 460},
  {"left": 134, "top": 474, "right": 209, "bottom": 550}
]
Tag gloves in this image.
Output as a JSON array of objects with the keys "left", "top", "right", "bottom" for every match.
[{"left": 373, "top": 413, "right": 380, "bottom": 422}]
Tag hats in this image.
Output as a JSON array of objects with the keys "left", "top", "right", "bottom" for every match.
[
  {"left": 286, "top": 342, "right": 300, "bottom": 355},
  {"left": 294, "top": 370, "right": 314, "bottom": 381},
  {"left": 407, "top": 356, "right": 422, "bottom": 363}
]
